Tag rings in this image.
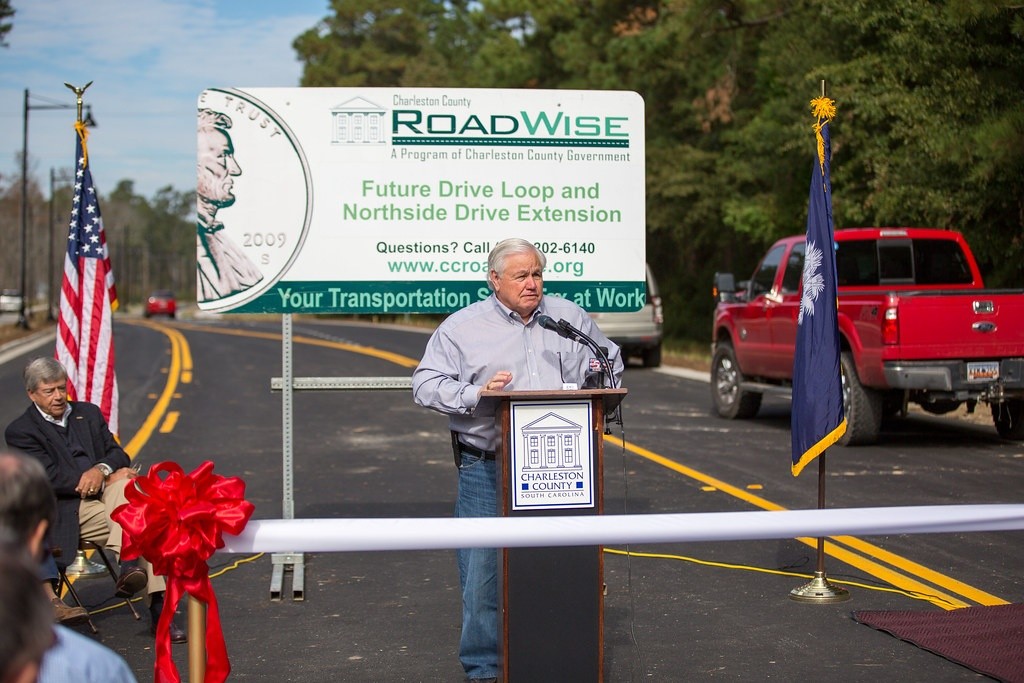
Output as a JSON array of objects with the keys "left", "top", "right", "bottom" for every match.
[{"left": 88, "top": 487, "right": 96, "bottom": 492}]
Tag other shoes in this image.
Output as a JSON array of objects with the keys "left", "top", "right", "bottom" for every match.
[{"left": 50, "top": 598, "right": 90, "bottom": 626}]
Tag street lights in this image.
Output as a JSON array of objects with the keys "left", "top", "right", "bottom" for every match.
[{"left": 13, "top": 89, "right": 97, "bottom": 330}]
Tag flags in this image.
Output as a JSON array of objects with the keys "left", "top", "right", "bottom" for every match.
[
  {"left": 791, "top": 97, "right": 846, "bottom": 477},
  {"left": 53, "top": 122, "right": 120, "bottom": 446}
]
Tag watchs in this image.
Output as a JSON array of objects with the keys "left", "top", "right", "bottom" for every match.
[{"left": 95, "top": 465, "right": 111, "bottom": 479}]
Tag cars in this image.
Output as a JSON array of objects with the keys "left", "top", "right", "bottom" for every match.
[
  {"left": 0, "top": 289, "right": 27, "bottom": 312},
  {"left": 587, "top": 261, "right": 664, "bottom": 368},
  {"left": 143, "top": 288, "right": 176, "bottom": 318}
]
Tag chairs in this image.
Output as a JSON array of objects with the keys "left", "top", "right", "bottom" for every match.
[{"left": 49, "top": 539, "right": 141, "bottom": 634}]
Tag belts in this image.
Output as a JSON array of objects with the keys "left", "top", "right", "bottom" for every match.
[{"left": 458, "top": 441, "right": 496, "bottom": 461}]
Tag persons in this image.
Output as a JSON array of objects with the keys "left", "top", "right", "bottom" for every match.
[
  {"left": 413, "top": 238, "right": 624, "bottom": 683},
  {"left": 0, "top": 445, "right": 138, "bottom": 683},
  {"left": 4, "top": 357, "right": 189, "bottom": 644}
]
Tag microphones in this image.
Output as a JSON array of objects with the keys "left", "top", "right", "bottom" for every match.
[
  {"left": 589, "top": 346, "right": 614, "bottom": 389},
  {"left": 538, "top": 316, "right": 588, "bottom": 346}
]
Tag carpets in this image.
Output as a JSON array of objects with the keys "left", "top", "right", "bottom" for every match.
[{"left": 850, "top": 602, "right": 1024, "bottom": 683}]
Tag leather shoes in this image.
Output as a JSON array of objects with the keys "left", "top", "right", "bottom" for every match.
[
  {"left": 114, "top": 565, "right": 148, "bottom": 598},
  {"left": 149, "top": 618, "right": 188, "bottom": 644}
]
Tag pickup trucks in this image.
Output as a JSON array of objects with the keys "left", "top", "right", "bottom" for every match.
[{"left": 711, "top": 227, "right": 1024, "bottom": 447}]
